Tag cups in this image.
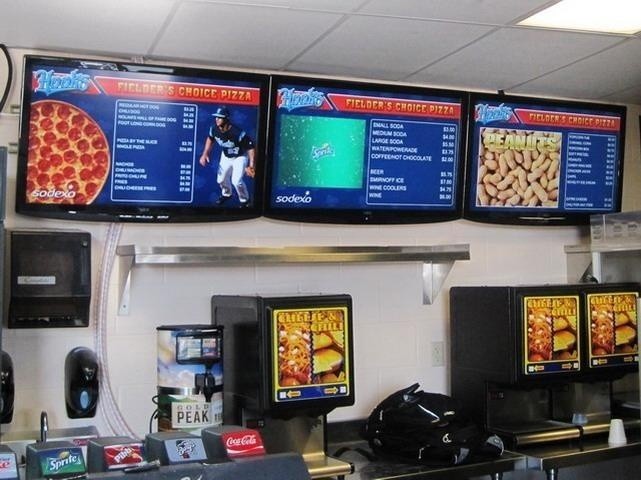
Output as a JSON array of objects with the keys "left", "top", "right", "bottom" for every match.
[{"left": 607, "top": 419, "right": 627, "bottom": 447}]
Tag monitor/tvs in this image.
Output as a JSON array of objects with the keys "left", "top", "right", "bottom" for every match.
[
  {"left": 463, "top": 92, "right": 627, "bottom": 226},
  {"left": 264, "top": 75, "right": 469, "bottom": 229},
  {"left": 17, "top": 55, "right": 270, "bottom": 224}
]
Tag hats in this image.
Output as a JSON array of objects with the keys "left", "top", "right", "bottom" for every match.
[{"left": 211, "top": 105, "right": 230, "bottom": 118}]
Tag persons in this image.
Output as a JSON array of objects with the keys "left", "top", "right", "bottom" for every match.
[{"left": 198, "top": 104, "right": 256, "bottom": 210}]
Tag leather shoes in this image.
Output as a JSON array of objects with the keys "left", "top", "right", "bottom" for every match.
[
  {"left": 216, "top": 194, "right": 232, "bottom": 204},
  {"left": 239, "top": 201, "right": 249, "bottom": 209}
]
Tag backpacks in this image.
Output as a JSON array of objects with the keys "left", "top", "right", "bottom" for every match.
[{"left": 332, "top": 380, "right": 504, "bottom": 467}]
